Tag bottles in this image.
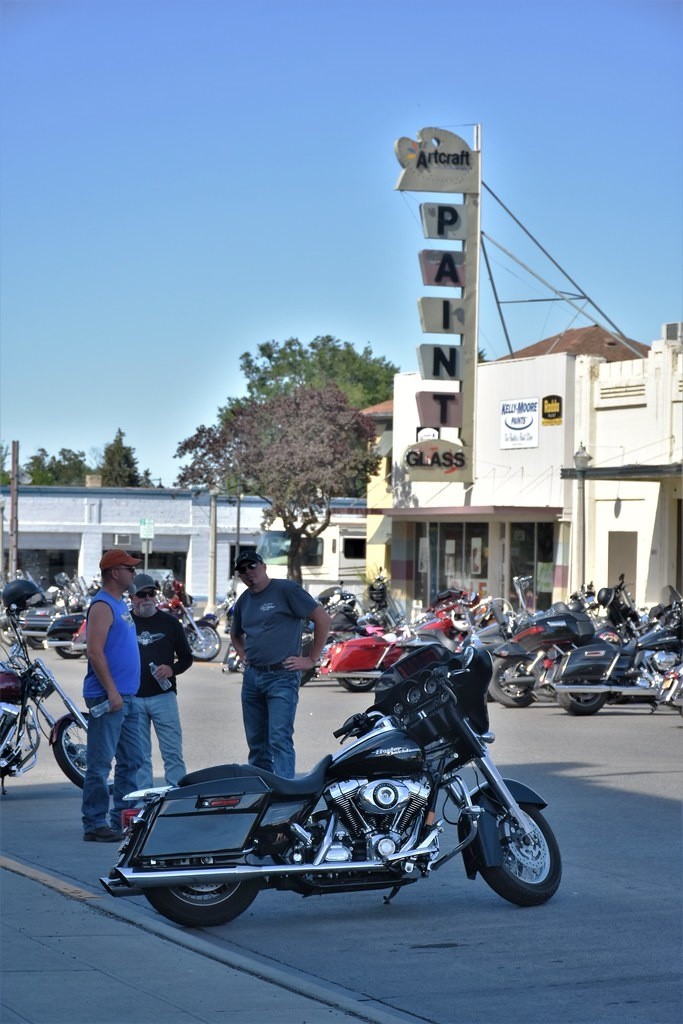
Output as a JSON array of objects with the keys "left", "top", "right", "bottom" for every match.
[
  {"left": 149, "top": 663, "right": 172, "bottom": 690},
  {"left": 90, "top": 700, "right": 111, "bottom": 717}
]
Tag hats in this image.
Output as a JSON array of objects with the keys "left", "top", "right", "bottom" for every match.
[
  {"left": 99, "top": 549, "right": 143, "bottom": 569},
  {"left": 128, "top": 573, "right": 157, "bottom": 595},
  {"left": 234, "top": 550, "right": 264, "bottom": 570}
]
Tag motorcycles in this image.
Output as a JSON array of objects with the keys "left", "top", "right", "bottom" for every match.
[
  {"left": 97, "top": 602, "right": 562, "bottom": 929},
  {"left": 0, "top": 568, "right": 683, "bottom": 716},
  {"left": 0, "top": 601, "right": 116, "bottom": 796}
]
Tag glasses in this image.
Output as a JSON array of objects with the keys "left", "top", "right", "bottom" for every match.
[
  {"left": 117, "top": 566, "right": 135, "bottom": 573},
  {"left": 135, "top": 591, "right": 156, "bottom": 599},
  {"left": 236, "top": 562, "right": 258, "bottom": 574}
]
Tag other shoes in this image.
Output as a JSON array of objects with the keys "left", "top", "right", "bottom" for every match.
[{"left": 84, "top": 826, "right": 123, "bottom": 842}]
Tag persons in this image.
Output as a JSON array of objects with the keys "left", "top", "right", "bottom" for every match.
[
  {"left": 81, "top": 549, "right": 144, "bottom": 843},
  {"left": 129, "top": 573, "right": 193, "bottom": 790},
  {"left": 230, "top": 551, "right": 332, "bottom": 779}
]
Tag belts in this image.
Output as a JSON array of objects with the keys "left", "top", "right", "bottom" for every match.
[{"left": 249, "top": 660, "right": 285, "bottom": 673}]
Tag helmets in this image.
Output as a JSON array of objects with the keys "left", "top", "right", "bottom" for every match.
[
  {"left": 598, "top": 588, "right": 615, "bottom": 609},
  {"left": 2, "top": 580, "right": 42, "bottom": 608},
  {"left": 369, "top": 583, "right": 386, "bottom": 603}
]
